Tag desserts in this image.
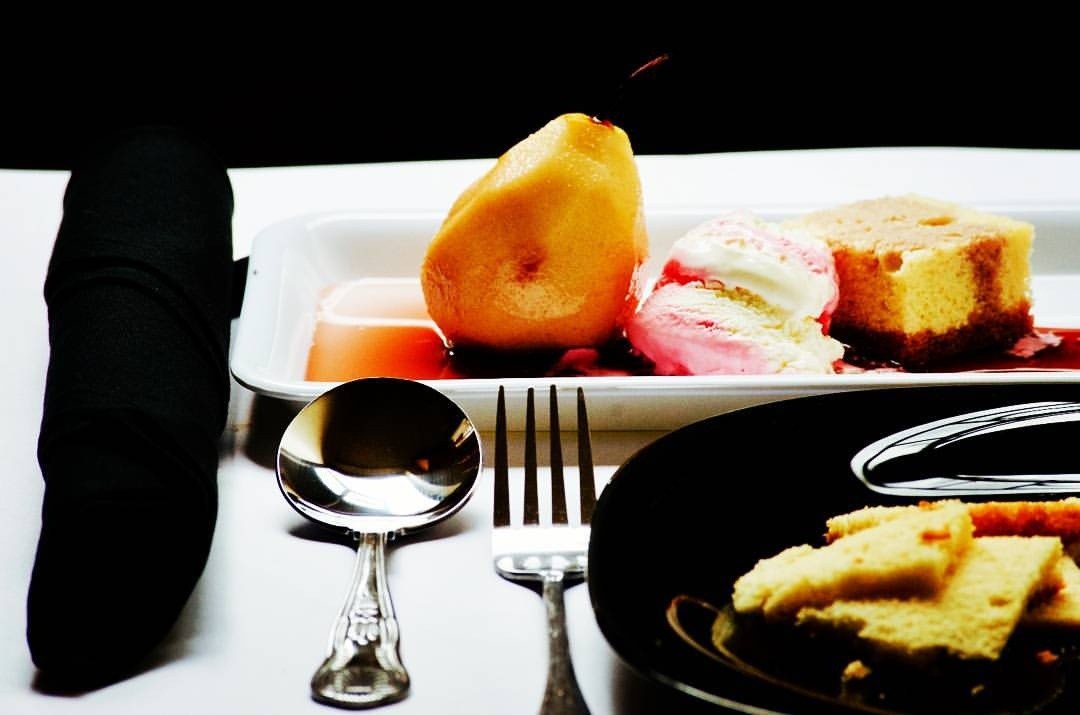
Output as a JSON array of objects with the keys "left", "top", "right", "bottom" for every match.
[{"left": 629, "top": 213, "right": 850, "bottom": 377}]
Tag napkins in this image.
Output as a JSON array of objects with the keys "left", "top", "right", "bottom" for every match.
[{"left": 28, "top": 108, "right": 250, "bottom": 699}]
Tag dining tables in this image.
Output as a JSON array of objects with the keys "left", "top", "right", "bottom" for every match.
[{"left": 0, "top": 148, "right": 1080, "bottom": 715}]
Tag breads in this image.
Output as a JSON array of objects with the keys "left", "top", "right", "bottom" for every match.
[
  {"left": 792, "top": 194, "right": 1038, "bottom": 372},
  {"left": 727, "top": 495, "right": 1080, "bottom": 667}
]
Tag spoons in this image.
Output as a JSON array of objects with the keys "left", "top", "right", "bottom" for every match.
[
  {"left": 276, "top": 378, "right": 482, "bottom": 712},
  {"left": 492, "top": 384, "right": 600, "bottom": 715}
]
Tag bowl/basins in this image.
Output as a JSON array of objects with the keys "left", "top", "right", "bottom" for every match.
[{"left": 589, "top": 384, "right": 1080, "bottom": 715}]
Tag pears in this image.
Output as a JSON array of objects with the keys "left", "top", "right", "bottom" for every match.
[{"left": 419, "top": 55, "right": 678, "bottom": 363}]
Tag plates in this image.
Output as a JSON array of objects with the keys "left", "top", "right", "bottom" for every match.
[{"left": 227, "top": 147, "right": 1080, "bottom": 430}]
